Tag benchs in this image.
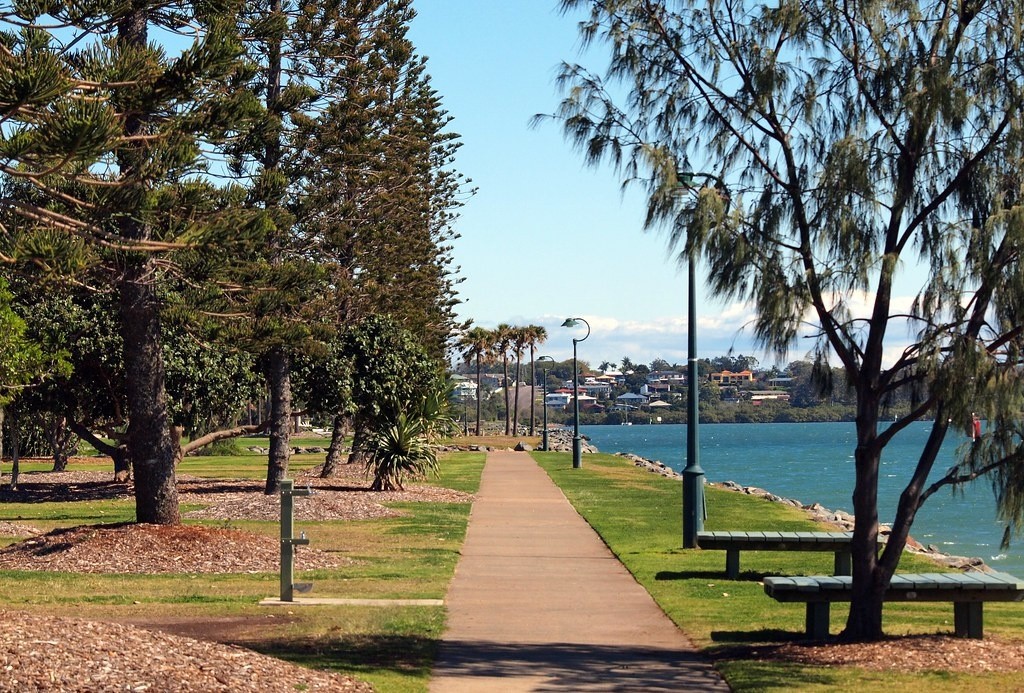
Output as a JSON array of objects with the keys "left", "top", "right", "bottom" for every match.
[
  {"left": 697, "top": 531, "right": 885, "bottom": 579},
  {"left": 763, "top": 571, "right": 1018, "bottom": 641}
]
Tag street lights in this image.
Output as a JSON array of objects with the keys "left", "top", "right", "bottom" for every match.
[
  {"left": 537, "top": 356, "right": 555, "bottom": 452},
  {"left": 677, "top": 172, "right": 731, "bottom": 550},
  {"left": 561, "top": 317, "right": 590, "bottom": 468}
]
